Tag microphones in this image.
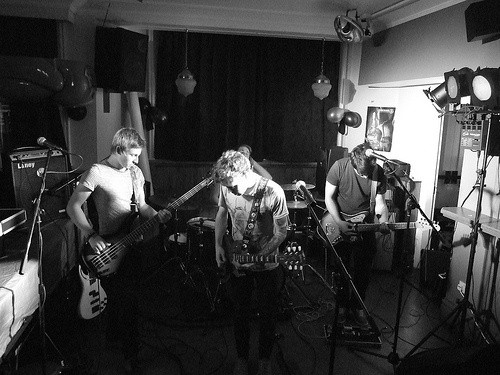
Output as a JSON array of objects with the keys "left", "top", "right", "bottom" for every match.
[
  {"left": 365, "top": 149, "right": 400, "bottom": 166},
  {"left": 37, "top": 136, "right": 69, "bottom": 155},
  {"left": 296, "top": 180, "right": 317, "bottom": 207}
]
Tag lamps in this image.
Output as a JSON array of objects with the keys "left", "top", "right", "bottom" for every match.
[
  {"left": 176, "top": 29, "right": 197, "bottom": 96},
  {"left": 311, "top": 39, "right": 332, "bottom": 99},
  {"left": 426, "top": 81, "right": 450, "bottom": 111},
  {"left": 471, "top": 66, "right": 500, "bottom": 105},
  {"left": 445, "top": 67, "right": 474, "bottom": 102}
]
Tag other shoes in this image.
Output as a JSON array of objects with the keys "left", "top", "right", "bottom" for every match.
[
  {"left": 234, "top": 358, "right": 249, "bottom": 375},
  {"left": 257, "top": 358, "right": 271, "bottom": 375}
]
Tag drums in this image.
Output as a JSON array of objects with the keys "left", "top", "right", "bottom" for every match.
[{"left": 186, "top": 216, "right": 216, "bottom": 268}]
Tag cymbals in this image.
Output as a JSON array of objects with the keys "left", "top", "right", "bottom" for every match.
[
  {"left": 286, "top": 200, "right": 308, "bottom": 209},
  {"left": 148, "top": 194, "right": 199, "bottom": 210},
  {"left": 281, "top": 183, "right": 316, "bottom": 190}
]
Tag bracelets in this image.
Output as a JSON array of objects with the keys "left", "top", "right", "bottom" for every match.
[{"left": 85, "top": 231, "right": 95, "bottom": 244}]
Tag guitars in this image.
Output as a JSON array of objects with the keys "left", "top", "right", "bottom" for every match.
[
  {"left": 195, "top": 230, "right": 307, "bottom": 272},
  {"left": 318, "top": 208, "right": 441, "bottom": 247},
  {"left": 77, "top": 174, "right": 216, "bottom": 281},
  {"left": 78, "top": 201, "right": 109, "bottom": 321}
]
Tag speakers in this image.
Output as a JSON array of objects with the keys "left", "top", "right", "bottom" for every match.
[
  {"left": 316, "top": 146, "right": 348, "bottom": 197},
  {"left": 94, "top": 25, "right": 149, "bottom": 92},
  {"left": 465, "top": 0, "right": 500, "bottom": 43},
  {"left": 2, "top": 155, "right": 71, "bottom": 231},
  {"left": 372, "top": 210, "right": 397, "bottom": 274},
  {"left": 4, "top": 216, "right": 78, "bottom": 297}
]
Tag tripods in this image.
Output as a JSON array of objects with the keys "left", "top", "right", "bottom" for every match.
[{"left": 402, "top": 111, "right": 500, "bottom": 357}]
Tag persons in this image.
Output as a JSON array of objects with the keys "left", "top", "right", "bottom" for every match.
[
  {"left": 212, "top": 147, "right": 289, "bottom": 375},
  {"left": 325, "top": 143, "right": 394, "bottom": 327},
  {"left": 66, "top": 129, "right": 171, "bottom": 372}
]
